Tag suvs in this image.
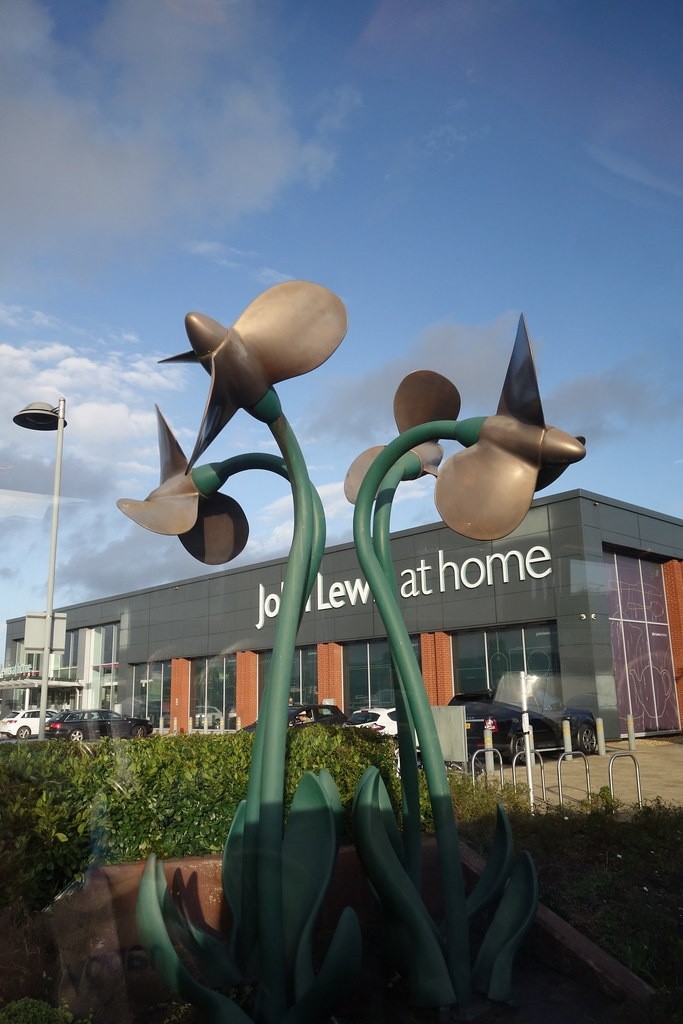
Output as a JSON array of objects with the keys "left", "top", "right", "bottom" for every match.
[
  {"left": 44, "top": 710, "right": 153, "bottom": 743},
  {"left": 447, "top": 687, "right": 598, "bottom": 766},
  {"left": 0, "top": 709, "right": 60, "bottom": 739}
]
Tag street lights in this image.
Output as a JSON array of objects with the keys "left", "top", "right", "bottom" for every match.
[{"left": 13, "top": 393, "right": 68, "bottom": 742}]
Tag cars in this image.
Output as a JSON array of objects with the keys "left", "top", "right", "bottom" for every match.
[
  {"left": 236, "top": 703, "right": 349, "bottom": 736},
  {"left": 342, "top": 707, "right": 420, "bottom": 765},
  {"left": 127, "top": 695, "right": 237, "bottom": 730},
  {"left": 349, "top": 688, "right": 395, "bottom": 715}
]
